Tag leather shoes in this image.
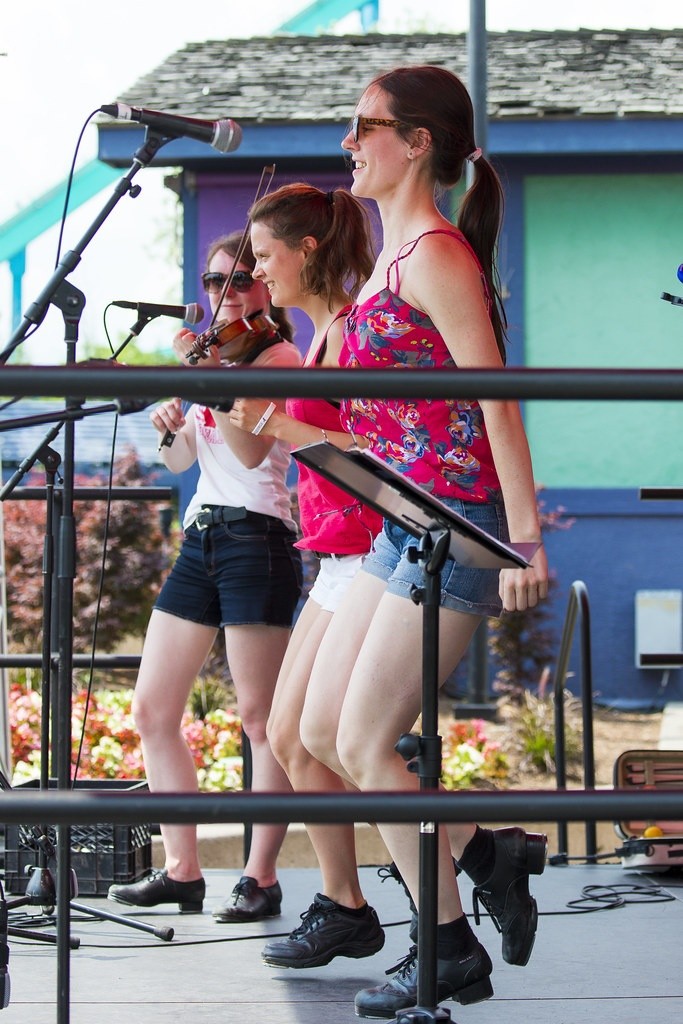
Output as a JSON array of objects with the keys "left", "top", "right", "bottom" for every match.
[
  {"left": 212, "top": 875, "right": 284, "bottom": 923},
  {"left": 107, "top": 867, "right": 206, "bottom": 914}
]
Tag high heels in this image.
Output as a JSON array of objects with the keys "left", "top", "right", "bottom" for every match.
[
  {"left": 354, "top": 939, "right": 495, "bottom": 1019},
  {"left": 472, "top": 826, "right": 548, "bottom": 967}
]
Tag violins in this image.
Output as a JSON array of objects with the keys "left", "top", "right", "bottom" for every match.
[{"left": 185, "top": 315, "right": 282, "bottom": 365}]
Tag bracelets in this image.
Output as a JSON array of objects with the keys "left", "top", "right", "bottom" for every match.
[{"left": 251, "top": 401, "right": 277, "bottom": 436}]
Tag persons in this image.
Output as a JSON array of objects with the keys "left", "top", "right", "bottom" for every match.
[
  {"left": 227, "top": 182, "right": 462, "bottom": 969},
  {"left": 299, "top": 66, "right": 549, "bottom": 1020},
  {"left": 107, "top": 230, "right": 303, "bottom": 925}
]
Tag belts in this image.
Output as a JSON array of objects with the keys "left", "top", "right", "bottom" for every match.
[
  {"left": 195, "top": 504, "right": 247, "bottom": 532},
  {"left": 312, "top": 550, "right": 348, "bottom": 561}
]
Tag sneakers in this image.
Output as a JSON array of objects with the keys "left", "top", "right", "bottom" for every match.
[
  {"left": 377, "top": 855, "right": 463, "bottom": 944},
  {"left": 261, "top": 892, "right": 385, "bottom": 970}
]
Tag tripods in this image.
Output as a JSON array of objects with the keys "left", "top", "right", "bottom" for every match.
[{"left": 3, "top": 124, "right": 178, "bottom": 940}]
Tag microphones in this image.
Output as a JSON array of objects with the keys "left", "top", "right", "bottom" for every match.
[
  {"left": 104, "top": 102, "right": 244, "bottom": 157},
  {"left": 111, "top": 301, "right": 205, "bottom": 325}
]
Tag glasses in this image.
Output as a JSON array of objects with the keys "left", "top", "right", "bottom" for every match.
[
  {"left": 353, "top": 115, "right": 415, "bottom": 143},
  {"left": 201, "top": 270, "right": 253, "bottom": 294}
]
